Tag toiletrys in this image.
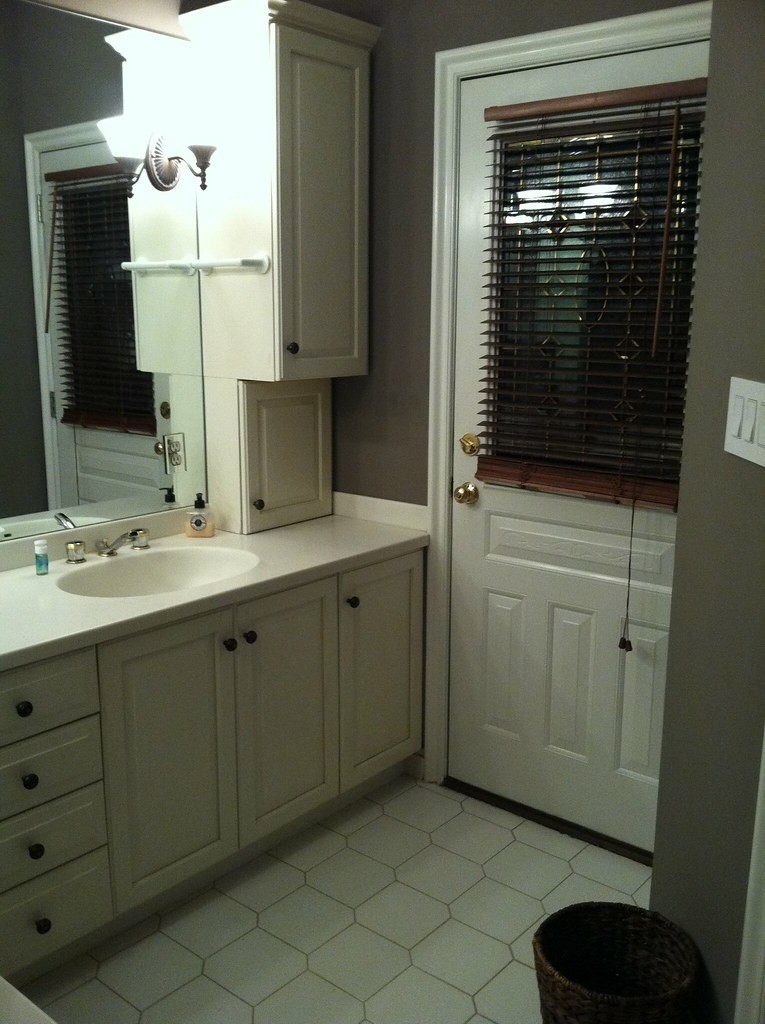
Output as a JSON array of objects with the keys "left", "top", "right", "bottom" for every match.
[
  {"left": 158, "top": 486, "right": 181, "bottom": 509},
  {"left": 185, "top": 492, "right": 215, "bottom": 539},
  {"left": 34, "top": 538, "right": 48, "bottom": 576}
]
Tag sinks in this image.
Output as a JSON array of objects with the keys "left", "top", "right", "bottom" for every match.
[
  {"left": 54, "top": 541, "right": 261, "bottom": 604},
  {"left": 0, "top": 515, "right": 111, "bottom": 540}
]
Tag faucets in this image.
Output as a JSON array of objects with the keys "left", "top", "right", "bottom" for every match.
[
  {"left": 95, "top": 527, "right": 150, "bottom": 556},
  {"left": 54, "top": 513, "right": 75, "bottom": 529}
]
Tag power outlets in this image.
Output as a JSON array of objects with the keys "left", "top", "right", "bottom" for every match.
[{"left": 163, "top": 432, "right": 188, "bottom": 475}]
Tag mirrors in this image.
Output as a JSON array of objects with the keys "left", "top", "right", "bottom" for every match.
[{"left": 0, "top": 0, "right": 210, "bottom": 545}]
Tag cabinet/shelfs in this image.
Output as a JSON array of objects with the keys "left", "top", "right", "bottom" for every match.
[
  {"left": 199, "top": 0, "right": 385, "bottom": 538},
  {"left": 0, "top": 546, "right": 428, "bottom": 991},
  {"left": 103, "top": 27, "right": 207, "bottom": 377}
]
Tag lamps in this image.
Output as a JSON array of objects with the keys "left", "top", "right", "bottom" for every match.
[
  {"left": 146, "top": 105, "right": 233, "bottom": 192},
  {"left": 96, "top": 113, "right": 163, "bottom": 199}
]
